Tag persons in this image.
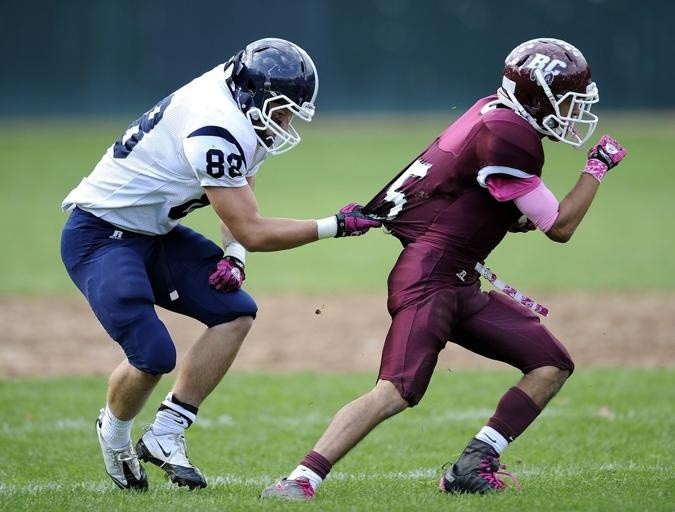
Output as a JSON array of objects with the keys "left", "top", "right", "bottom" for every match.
[{"left": 60, "top": 37, "right": 382, "bottom": 494}]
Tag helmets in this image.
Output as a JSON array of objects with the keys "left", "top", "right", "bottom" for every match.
[
  {"left": 500, "top": 36, "right": 602, "bottom": 148},
  {"left": 227, "top": 35, "right": 320, "bottom": 156}
]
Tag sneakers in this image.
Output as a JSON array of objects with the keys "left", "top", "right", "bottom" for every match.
[
  {"left": 440, "top": 456, "right": 519, "bottom": 495},
  {"left": 95, "top": 409, "right": 148, "bottom": 490},
  {"left": 136, "top": 426, "right": 206, "bottom": 488},
  {"left": 261, "top": 477, "right": 317, "bottom": 500}
]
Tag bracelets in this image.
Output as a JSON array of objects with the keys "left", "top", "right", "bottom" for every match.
[
  {"left": 223, "top": 242, "right": 248, "bottom": 264},
  {"left": 317, "top": 218, "right": 339, "bottom": 240},
  {"left": 580, "top": 159, "right": 608, "bottom": 181},
  {"left": 258, "top": 38, "right": 628, "bottom": 502}
]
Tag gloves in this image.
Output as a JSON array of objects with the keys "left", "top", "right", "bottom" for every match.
[
  {"left": 209, "top": 256, "right": 246, "bottom": 293},
  {"left": 334, "top": 201, "right": 380, "bottom": 238},
  {"left": 583, "top": 130, "right": 630, "bottom": 178}
]
